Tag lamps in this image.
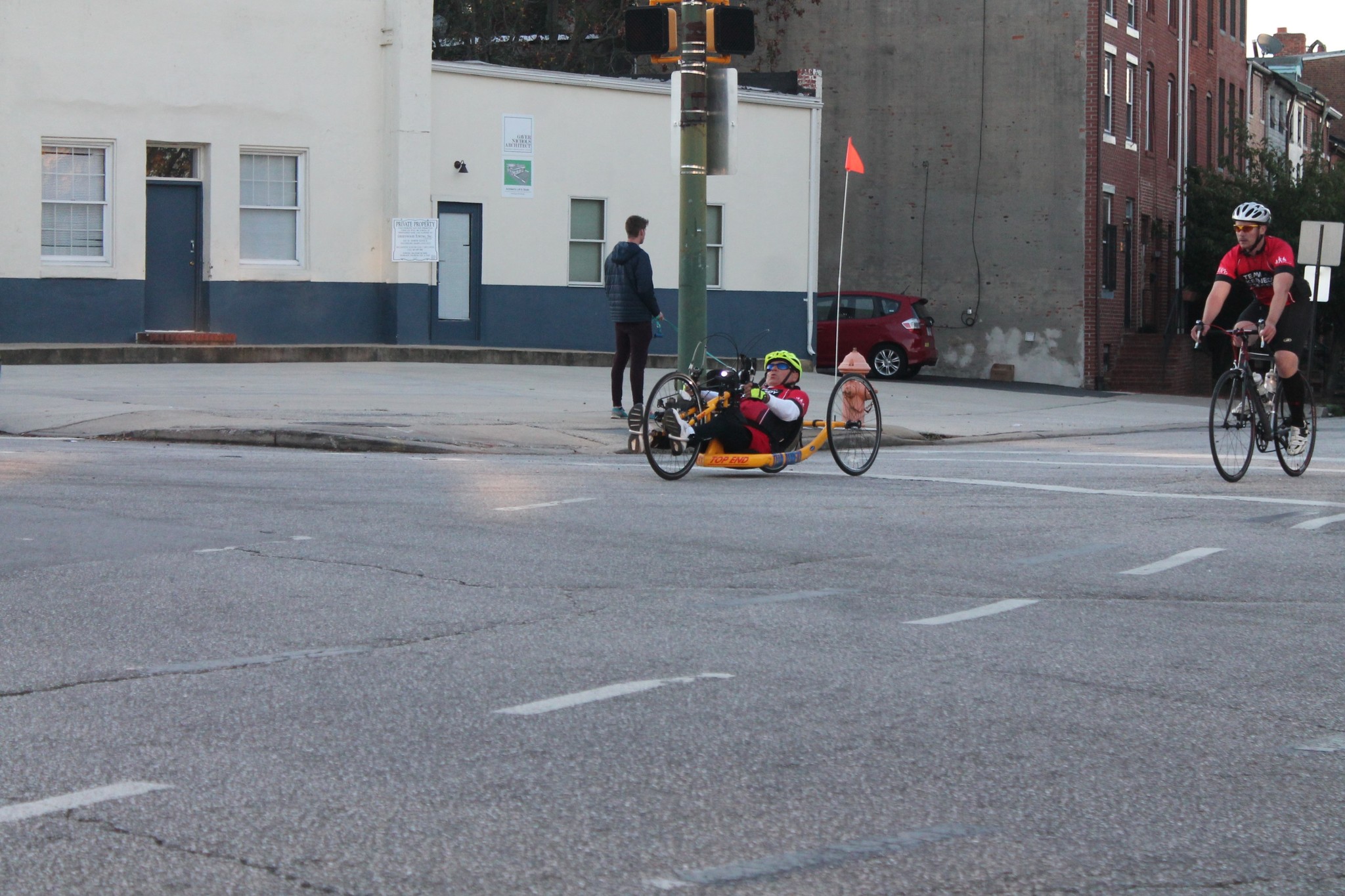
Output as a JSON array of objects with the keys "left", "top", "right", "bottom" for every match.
[{"left": 455, "top": 160, "right": 468, "bottom": 173}]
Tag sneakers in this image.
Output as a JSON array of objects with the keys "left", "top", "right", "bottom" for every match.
[
  {"left": 627, "top": 403, "right": 646, "bottom": 453},
  {"left": 1287, "top": 420, "right": 1309, "bottom": 456},
  {"left": 1231, "top": 395, "right": 1269, "bottom": 418},
  {"left": 649, "top": 411, "right": 656, "bottom": 423},
  {"left": 611, "top": 407, "right": 628, "bottom": 419},
  {"left": 663, "top": 408, "right": 689, "bottom": 456}
]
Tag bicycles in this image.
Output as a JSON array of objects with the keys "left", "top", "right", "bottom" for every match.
[{"left": 1194, "top": 319, "right": 1317, "bottom": 483}]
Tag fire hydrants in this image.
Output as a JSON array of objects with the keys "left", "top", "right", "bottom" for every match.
[{"left": 838, "top": 347, "right": 878, "bottom": 424}]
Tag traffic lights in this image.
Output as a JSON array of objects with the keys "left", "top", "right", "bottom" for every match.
[
  {"left": 626, "top": 2, "right": 678, "bottom": 55},
  {"left": 707, "top": 2, "right": 756, "bottom": 56}
]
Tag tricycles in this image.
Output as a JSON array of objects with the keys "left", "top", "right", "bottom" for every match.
[{"left": 629, "top": 328, "right": 883, "bottom": 480}]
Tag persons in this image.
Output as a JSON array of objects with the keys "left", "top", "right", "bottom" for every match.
[
  {"left": 628, "top": 350, "right": 809, "bottom": 454},
  {"left": 1190, "top": 202, "right": 1313, "bottom": 455},
  {"left": 604, "top": 215, "right": 664, "bottom": 419}
]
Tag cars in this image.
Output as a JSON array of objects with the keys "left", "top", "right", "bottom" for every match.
[{"left": 817, "top": 291, "right": 939, "bottom": 380}]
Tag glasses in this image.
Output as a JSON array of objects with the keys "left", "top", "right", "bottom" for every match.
[
  {"left": 766, "top": 363, "right": 792, "bottom": 371},
  {"left": 1232, "top": 224, "right": 1262, "bottom": 233}
]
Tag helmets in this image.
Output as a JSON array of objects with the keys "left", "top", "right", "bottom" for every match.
[
  {"left": 764, "top": 350, "right": 802, "bottom": 382},
  {"left": 1232, "top": 201, "right": 1272, "bottom": 224}
]
